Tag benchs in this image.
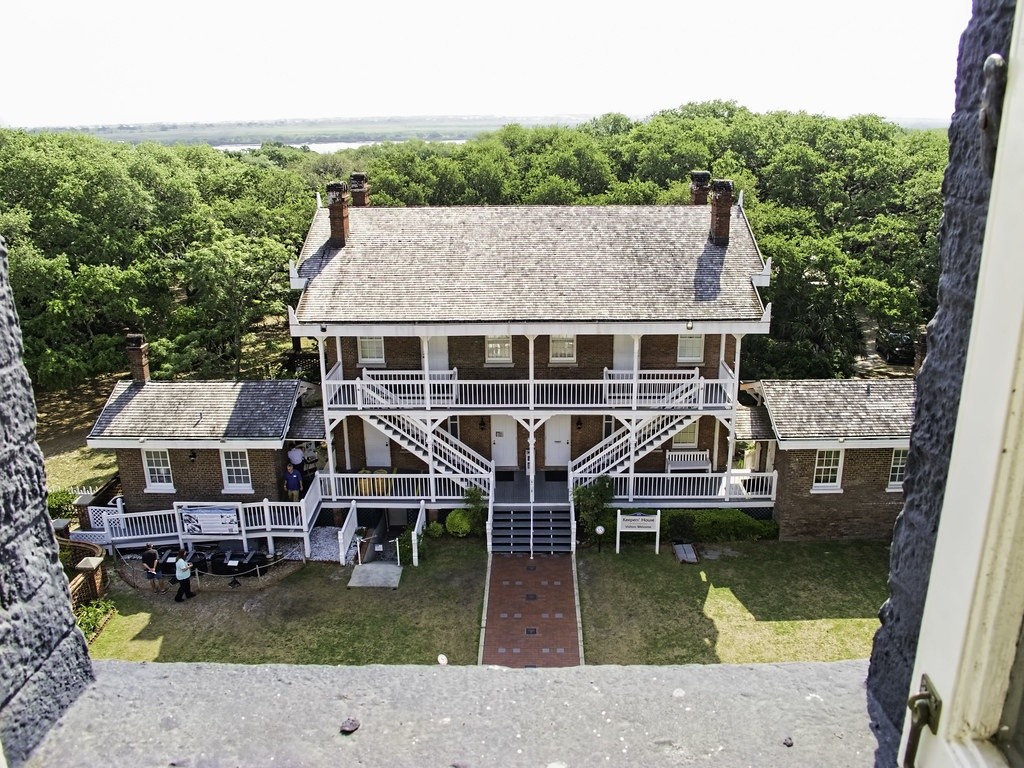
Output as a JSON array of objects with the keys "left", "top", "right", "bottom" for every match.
[{"left": 665, "top": 449, "right": 711, "bottom": 479}]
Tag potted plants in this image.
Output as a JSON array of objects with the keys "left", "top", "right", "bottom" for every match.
[{"left": 352, "top": 453, "right": 373, "bottom": 496}]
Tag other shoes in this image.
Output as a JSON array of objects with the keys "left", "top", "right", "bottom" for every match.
[
  {"left": 175, "top": 597, "right": 184, "bottom": 602},
  {"left": 186, "top": 592, "right": 197, "bottom": 598}
]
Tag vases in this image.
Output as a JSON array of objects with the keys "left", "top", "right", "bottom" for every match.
[{"left": 373, "top": 467, "right": 388, "bottom": 494}]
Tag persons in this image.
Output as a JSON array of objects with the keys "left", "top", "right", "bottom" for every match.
[
  {"left": 142, "top": 542, "right": 170, "bottom": 595},
  {"left": 283, "top": 464, "right": 303, "bottom": 502},
  {"left": 287, "top": 443, "right": 305, "bottom": 475},
  {"left": 175, "top": 549, "right": 197, "bottom": 602}
]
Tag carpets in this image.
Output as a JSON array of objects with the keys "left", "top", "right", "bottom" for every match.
[
  {"left": 495, "top": 471, "right": 515, "bottom": 481},
  {"left": 545, "top": 471, "right": 568, "bottom": 482},
  {"left": 744, "top": 478, "right": 768, "bottom": 493}
]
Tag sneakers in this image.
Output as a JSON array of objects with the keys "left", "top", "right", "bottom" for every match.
[
  {"left": 161, "top": 587, "right": 170, "bottom": 595},
  {"left": 154, "top": 587, "right": 159, "bottom": 594}
]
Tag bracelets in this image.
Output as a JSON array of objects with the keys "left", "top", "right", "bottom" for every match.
[{"left": 284, "top": 486, "right": 286, "bottom": 487}]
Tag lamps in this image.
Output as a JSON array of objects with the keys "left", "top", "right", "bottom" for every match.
[
  {"left": 478, "top": 417, "right": 486, "bottom": 431},
  {"left": 595, "top": 526, "right": 605, "bottom": 535},
  {"left": 576, "top": 417, "right": 583, "bottom": 430}
]
